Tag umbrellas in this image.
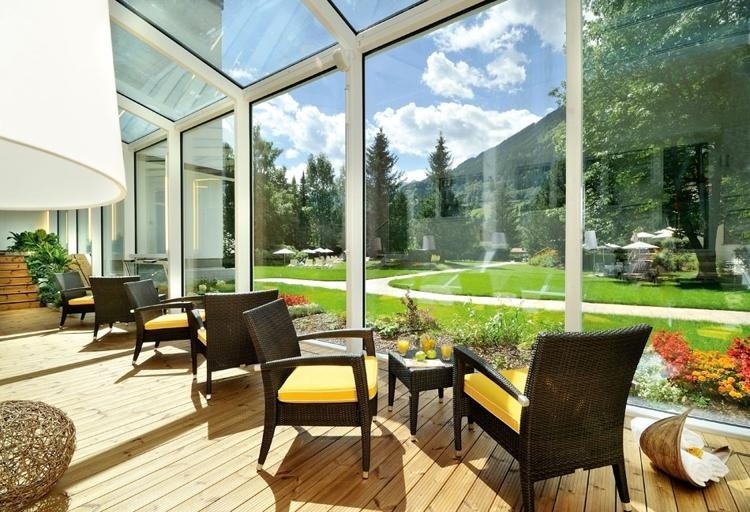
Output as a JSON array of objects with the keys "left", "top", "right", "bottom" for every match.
[
  {"left": 621, "top": 226, "right": 683, "bottom": 272},
  {"left": 273, "top": 247, "right": 295, "bottom": 265},
  {"left": 301, "top": 247, "right": 334, "bottom": 257}
]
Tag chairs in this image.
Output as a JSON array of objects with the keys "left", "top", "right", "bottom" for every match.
[
  {"left": 242, "top": 298, "right": 379, "bottom": 479},
  {"left": 123, "top": 280, "right": 206, "bottom": 375},
  {"left": 191, "top": 290, "right": 278, "bottom": 401},
  {"left": 452, "top": 323, "right": 654, "bottom": 512},
  {"left": 52, "top": 271, "right": 94, "bottom": 331},
  {"left": 593, "top": 259, "right": 660, "bottom": 285},
  {"left": 288, "top": 255, "right": 344, "bottom": 270},
  {"left": 89, "top": 275, "right": 141, "bottom": 338}
]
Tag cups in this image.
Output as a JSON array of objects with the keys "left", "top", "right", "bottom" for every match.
[
  {"left": 398, "top": 336, "right": 410, "bottom": 357},
  {"left": 440, "top": 342, "right": 453, "bottom": 362}
]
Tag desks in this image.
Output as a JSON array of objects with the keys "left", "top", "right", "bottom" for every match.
[{"left": 386, "top": 347, "right": 475, "bottom": 441}]
